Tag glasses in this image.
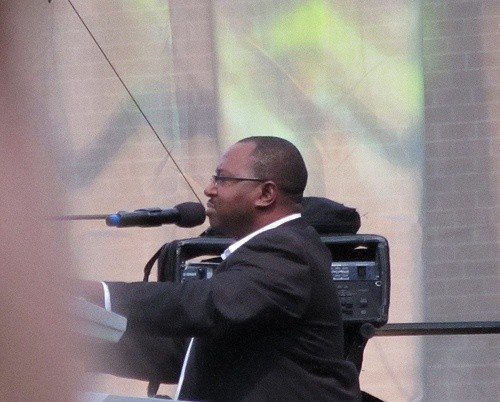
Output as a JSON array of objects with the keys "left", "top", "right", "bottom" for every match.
[{"left": 210, "top": 174, "right": 269, "bottom": 186}]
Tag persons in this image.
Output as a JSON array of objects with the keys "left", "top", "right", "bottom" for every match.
[{"left": 69, "top": 135, "right": 363, "bottom": 402}]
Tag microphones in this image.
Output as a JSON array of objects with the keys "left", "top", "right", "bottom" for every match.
[{"left": 104, "top": 202, "right": 206, "bottom": 229}]
